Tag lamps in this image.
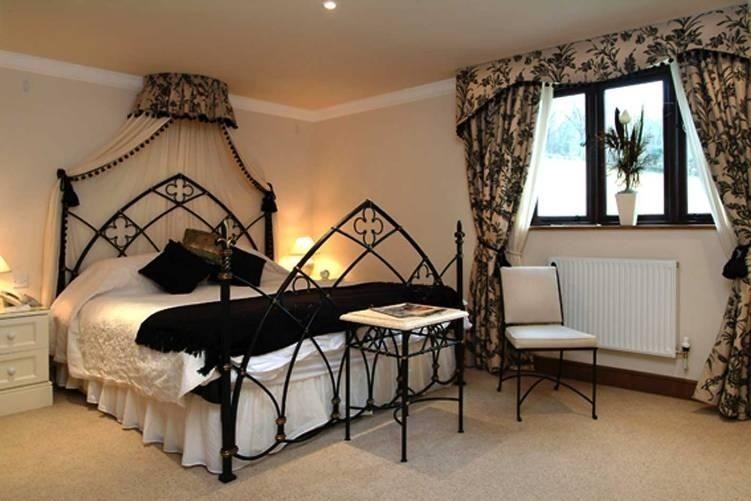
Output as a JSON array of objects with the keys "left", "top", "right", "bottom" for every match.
[
  {"left": 290, "top": 237, "right": 321, "bottom": 276},
  {"left": 0, "top": 256, "right": 12, "bottom": 272}
]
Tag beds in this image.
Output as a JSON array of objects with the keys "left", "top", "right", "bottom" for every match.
[{"left": 50, "top": 169, "right": 466, "bottom": 483}]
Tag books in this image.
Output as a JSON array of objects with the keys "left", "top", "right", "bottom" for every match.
[{"left": 368, "top": 303, "right": 449, "bottom": 319}]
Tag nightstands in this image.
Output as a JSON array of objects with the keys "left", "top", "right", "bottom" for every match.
[{"left": 0, "top": 306, "right": 54, "bottom": 416}]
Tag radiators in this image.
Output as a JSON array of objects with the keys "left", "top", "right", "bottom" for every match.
[{"left": 548, "top": 258, "right": 690, "bottom": 369}]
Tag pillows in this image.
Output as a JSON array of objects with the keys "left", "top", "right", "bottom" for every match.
[{"left": 137, "top": 229, "right": 267, "bottom": 294}]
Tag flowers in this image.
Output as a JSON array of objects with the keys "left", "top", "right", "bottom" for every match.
[{"left": 580, "top": 104, "right": 655, "bottom": 191}]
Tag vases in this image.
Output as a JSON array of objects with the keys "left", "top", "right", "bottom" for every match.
[{"left": 615, "top": 191, "right": 640, "bottom": 225}]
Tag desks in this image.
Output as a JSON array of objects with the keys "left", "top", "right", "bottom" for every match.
[{"left": 329, "top": 300, "right": 469, "bottom": 462}]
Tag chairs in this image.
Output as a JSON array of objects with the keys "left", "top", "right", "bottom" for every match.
[{"left": 495, "top": 261, "right": 599, "bottom": 421}]
